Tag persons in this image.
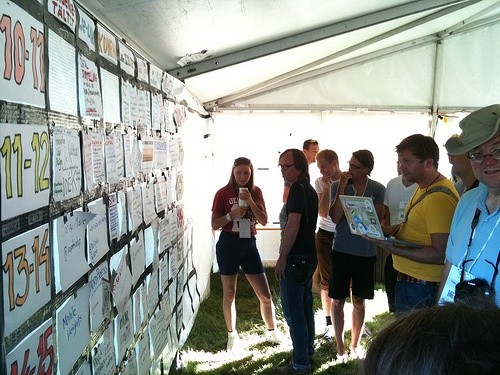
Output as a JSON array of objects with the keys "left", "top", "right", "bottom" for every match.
[
  {"left": 328, "top": 150, "right": 385, "bottom": 362},
  {"left": 315, "top": 149, "right": 344, "bottom": 339},
  {"left": 211, "top": 157, "right": 285, "bottom": 354},
  {"left": 274, "top": 149, "right": 319, "bottom": 375},
  {"left": 362, "top": 134, "right": 479, "bottom": 319},
  {"left": 362, "top": 296, "right": 500, "bottom": 375},
  {"left": 435, "top": 104, "right": 500, "bottom": 311},
  {"left": 282, "top": 139, "right": 320, "bottom": 203}
]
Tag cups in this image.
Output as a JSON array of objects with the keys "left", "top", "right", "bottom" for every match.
[{"left": 239, "top": 188, "right": 249, "bottom": 207}]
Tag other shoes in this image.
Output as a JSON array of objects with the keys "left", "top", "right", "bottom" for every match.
[
  {"left": 226, "top": 330, "right": 240, "bottom": 353},
  {"left": 265, "top": 329, "right": 289, "bottom": 345},
  {"left": 323, "top": 325, "right": 335, "bottom": 340}
]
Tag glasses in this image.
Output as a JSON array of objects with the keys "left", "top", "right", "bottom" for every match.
[
  {"left": 467, "top": 149, "right": 500, "bottom": 163},
  {"left": 346, "top": 160, "right": 365, "bottom": 170},
  {"left": 277, "top": 164, "right": 295, "bottom": 172}
]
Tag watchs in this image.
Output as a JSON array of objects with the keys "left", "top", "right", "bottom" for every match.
[{"left": 225, "top": 213, "right": 232, "bottom": 221}]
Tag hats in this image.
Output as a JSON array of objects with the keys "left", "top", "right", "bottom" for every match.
[{"left": 444, "top": 103, "right": 500, "bottom": 156}]
